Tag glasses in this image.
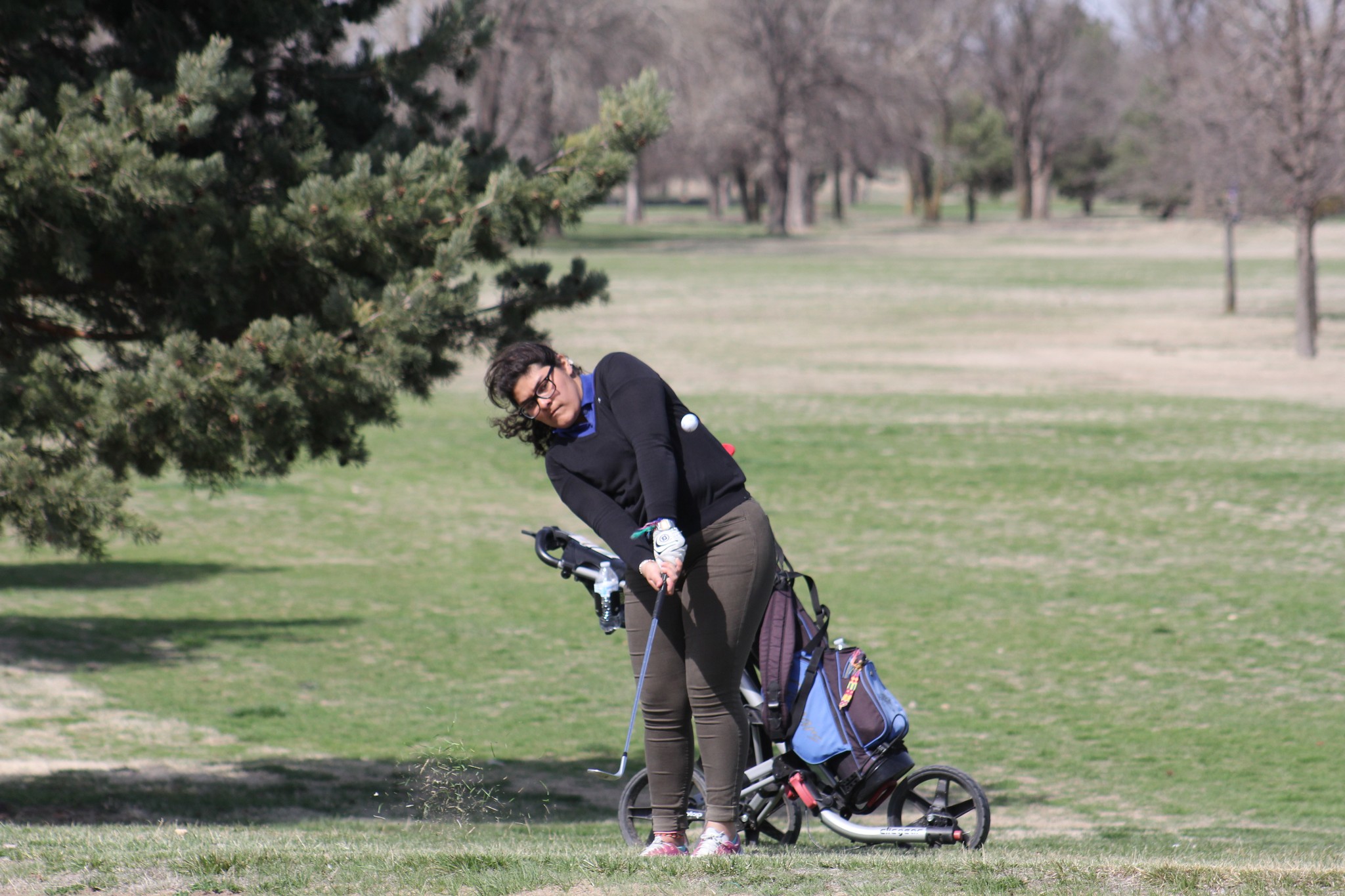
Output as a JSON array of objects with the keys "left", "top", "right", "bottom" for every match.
[{"left": 518, "top": 364, "right": 556, "bottom": 419}]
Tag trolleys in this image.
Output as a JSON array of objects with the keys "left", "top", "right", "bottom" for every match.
[{"left": 523, "top": 526, "right": 990, "bottom": 854}]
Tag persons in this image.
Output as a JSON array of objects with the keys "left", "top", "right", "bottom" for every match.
[{"left": 486, "top": 342, "right": 781, "bottom": 862}]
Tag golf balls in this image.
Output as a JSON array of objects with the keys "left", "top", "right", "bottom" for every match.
[{"left": 681, "top": 414, "right": 699, "bottom": 433}]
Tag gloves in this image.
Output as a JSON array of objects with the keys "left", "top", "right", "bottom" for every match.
[{"left": 653, "top": 519, "right": 688, "bottom": 574}]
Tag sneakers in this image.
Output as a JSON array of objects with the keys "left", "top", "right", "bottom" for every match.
[
  {"left": 690, "top": 827, "right": 744, "bottom": 858},
  {"left": 638, "top": 836, "right": 690, "bottom": 857}
]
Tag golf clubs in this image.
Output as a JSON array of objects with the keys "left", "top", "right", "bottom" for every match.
[{"left": 587, "top": 573, "right": 668, "bottom": 781}]
